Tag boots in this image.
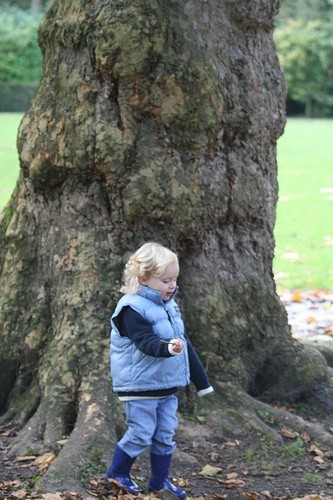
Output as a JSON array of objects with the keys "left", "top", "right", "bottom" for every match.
[
  {"left": 103, "top": 444, "right": 145, "bottom": 495},
  {"left": 147, "top": 452, "right": 187, "bottom": 500}
]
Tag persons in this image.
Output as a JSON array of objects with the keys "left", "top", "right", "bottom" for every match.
[{"left": 108, "top": 243, "right": 215, "bottom": 498}]
